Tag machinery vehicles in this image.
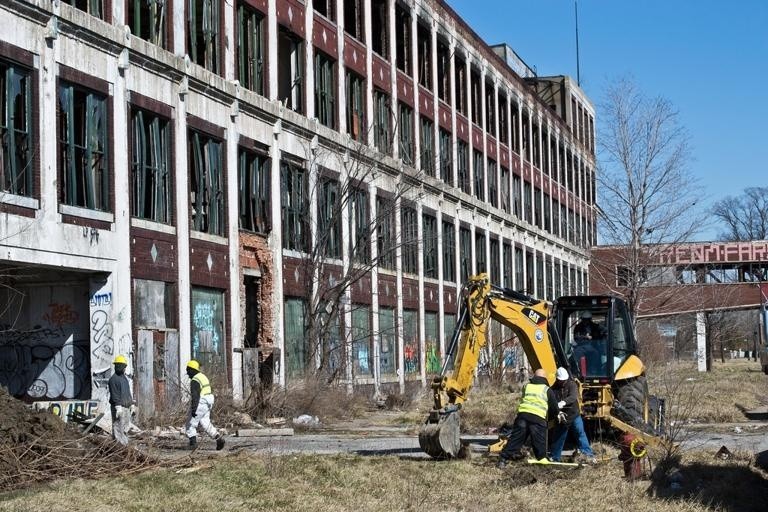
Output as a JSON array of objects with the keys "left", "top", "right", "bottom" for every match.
[{"left": 417, "top": 270, "right": 672, "bottom": 466}]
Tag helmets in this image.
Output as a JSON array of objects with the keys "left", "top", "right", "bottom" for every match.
[
  {"left": 112, "top": 356, "right": 127, "bottom": 366},
  {"left": 534, "top": 368, "right": 546, "bottom": 377},
  {"left": 186, "top": 360, "right": 199, "bottom": 371},
  {"left": 581, "top": 310, "right": 593, "bottom": 320},
  {"left": 556, "top": 367, "right": 569, "bottom": 381}
]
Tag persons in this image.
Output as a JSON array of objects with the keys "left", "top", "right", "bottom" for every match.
[
  {"left": 497, "top": 367, "right": 567, "bottom": 470},
  {"left": 548, "top": 367, "right": 596, "bottom": 464},
  {"left": 570, "top": 310, "right": 606, "bottom": 376},
  {"left": 186, "top": 360, "right": 225, "bottom": 452},
  {"left": 109, "top": 356, "right": 136, "bottom": 447}
]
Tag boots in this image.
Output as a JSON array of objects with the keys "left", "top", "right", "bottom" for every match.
[
  {"left": 184, "top": 436, "right": 197, "bottom": 450},
  {"left": 215, "top": 435, "right": 224, "bottom": 450}
]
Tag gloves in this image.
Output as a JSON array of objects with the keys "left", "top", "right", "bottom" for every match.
[
  {"left": 130, "top": 405, "right": 136, "bottom": 416},
  {"left": 557, "top": 411, "right": 567, "bottom": 424},
  {"left": 191, "top": 410, "right": 197, "bottom": 419},
  {"left": 115, "top": 405, "right": 123, "bottom": 419},
  {"left": 557, "top": 401, "right": 566, "bottom": 409}
]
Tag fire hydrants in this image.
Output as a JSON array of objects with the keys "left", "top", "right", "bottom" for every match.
[{"left": 617, "top": 429, "right": 647, "bottom": 480}]
